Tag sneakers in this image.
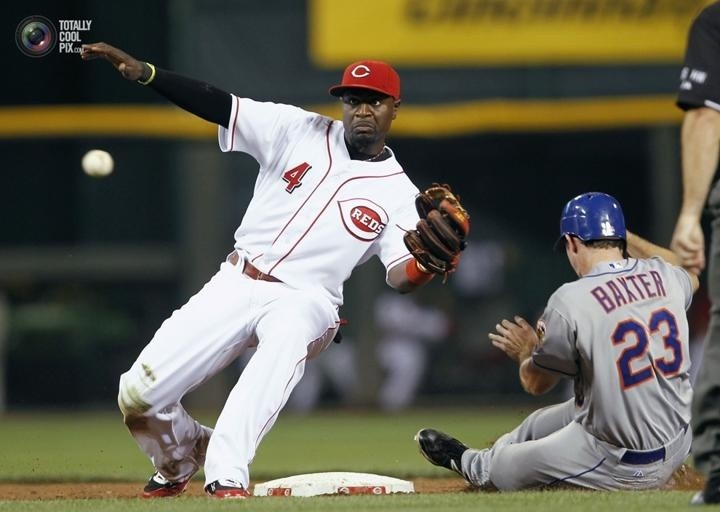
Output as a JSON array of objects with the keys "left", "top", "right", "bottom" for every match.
[
  {"left": 417, "top": 428, "right": 472, "bottom": 483},
  {"left": 141, "top": 426, "right": 214, "bottom": 498},
  {"left": 204, "top": 479, "right": 248, "bottom": 500},
  {"left": 692, "top": 474, "right": 719, "bottom": 506}
]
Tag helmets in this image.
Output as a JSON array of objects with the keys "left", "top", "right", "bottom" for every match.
[{"left": 553, "top": 192, "right": 626, "bottom": 248}]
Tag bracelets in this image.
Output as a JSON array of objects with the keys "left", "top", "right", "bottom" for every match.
[{"left": 136, "top": 61, "right": 156, "bottom": 85}]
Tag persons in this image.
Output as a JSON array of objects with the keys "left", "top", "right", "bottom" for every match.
[
  {"left": 669, "top": 1, "right": 720, "bottom": 506},
  {"left": 292, "top": 281, "right": 449, "bottom": 409},
  {"left": 78, "top": 41, "right": 473, "bottom": 497},
  {"left": 412, "top": 190, "right": 701, "bottom": 495}
]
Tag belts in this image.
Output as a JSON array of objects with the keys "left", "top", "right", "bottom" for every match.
[
  {"left": 622, "top": 447, "right": 664, "bottom": 465},
  {"left": 229, "top": 250, "right": 277, "bottom": 282}
]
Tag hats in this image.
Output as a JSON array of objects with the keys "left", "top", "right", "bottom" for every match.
[{"left": 329, "top": 60, "right": 400, "bottom": 101}]
